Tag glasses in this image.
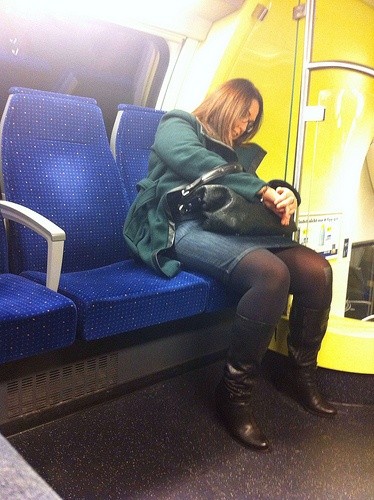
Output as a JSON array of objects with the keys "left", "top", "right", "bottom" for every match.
[{"left": 239, "top": 110, "right": 254, "bottom": 133}]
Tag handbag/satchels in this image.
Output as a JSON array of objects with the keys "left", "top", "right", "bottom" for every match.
[{"left": 178, "top": 164, "right": 297, "bottom": 236}]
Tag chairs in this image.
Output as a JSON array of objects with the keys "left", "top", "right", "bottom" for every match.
[{"left": 0, "top": 87, "right": 238, "bottom": 361}]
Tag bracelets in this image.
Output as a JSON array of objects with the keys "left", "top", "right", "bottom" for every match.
[{"left": 259, "top": 185, "right": 269, "bottom": 203}]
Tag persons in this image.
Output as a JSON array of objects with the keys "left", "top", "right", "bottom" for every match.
[{"left": 121, "top": 77, "right": 338, "bottom": 452}]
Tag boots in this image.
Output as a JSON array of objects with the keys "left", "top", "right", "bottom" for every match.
[
  {"left": 278, "top": 305, "right": 338, "bottom": 417},
  {"left": 216, "top": 313, "right": 271, "bottom": 452}
]
